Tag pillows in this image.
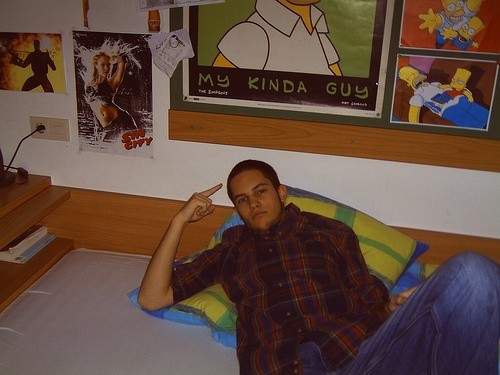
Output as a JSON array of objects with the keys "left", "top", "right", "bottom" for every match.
[{"left": 280, "top": 184, "right": 430, "bottom": 294}]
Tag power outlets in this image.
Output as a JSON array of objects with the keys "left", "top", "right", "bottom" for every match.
[{"left": 30, "top": 116, "right": 50, "bottom": 140}]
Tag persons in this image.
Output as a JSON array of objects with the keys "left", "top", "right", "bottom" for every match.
[
  {"left": 138, "top": 159, "right": 500, "bottom": 375},
  {"left": 73, "top": 40, "right": 142, "bottom": 141}
]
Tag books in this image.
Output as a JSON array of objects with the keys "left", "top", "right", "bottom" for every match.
[{"left": 0, "top": 223, "right": 56, "bottom": 265}]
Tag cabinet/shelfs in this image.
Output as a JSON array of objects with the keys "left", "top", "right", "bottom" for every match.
[{"left": 0, "top": 171, "right": 75, "bottom": 312}]
